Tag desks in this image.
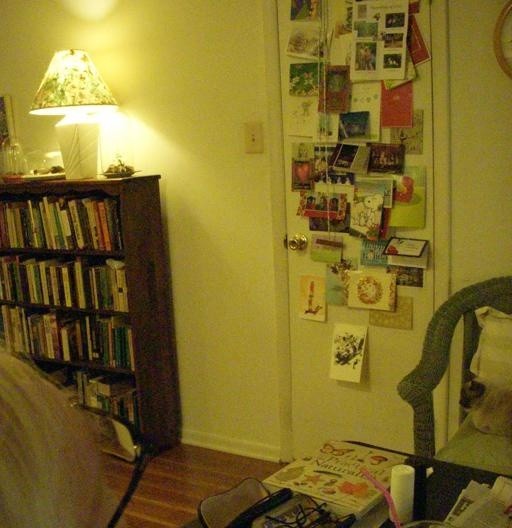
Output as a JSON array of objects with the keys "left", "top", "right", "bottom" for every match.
[{"left": 179, "top": 437, "right": 512, "bottom": 528}]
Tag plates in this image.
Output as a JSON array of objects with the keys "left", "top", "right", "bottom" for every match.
[
  {"left": 20, "top": 171, "right": 65, "bottom": 182},
  {"left": 97, "top": 168, "right": 145, "bottom": 178}
]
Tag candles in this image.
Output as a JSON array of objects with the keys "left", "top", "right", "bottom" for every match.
[{"left": 390, "top": 463, "right": 415, "bottom": 525}]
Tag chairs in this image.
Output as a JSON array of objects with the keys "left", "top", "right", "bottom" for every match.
[{"left": 395, "top": 274, "right": 512, "bottom": 475}]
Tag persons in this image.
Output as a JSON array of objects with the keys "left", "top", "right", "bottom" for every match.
[
  {"left": 363, "top": 45, "right": 373, "bottom": 70},
  {"left": 359, "top": 43, "right": 366, "bottom": 70},
  {"left": 306, "top": 194, "right": 339, "bottom": 212}
]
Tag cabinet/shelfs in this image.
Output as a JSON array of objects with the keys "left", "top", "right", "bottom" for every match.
[{"left": 0, "top": 175, "right": 183, "bottom": 453}]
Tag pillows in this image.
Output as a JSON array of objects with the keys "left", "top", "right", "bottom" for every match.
[
  {"left": 471, "top": 379, "right": 512, "bottom": 437},
  {"left": 469, "top": 302, "right": 512, "bottom": 386}
]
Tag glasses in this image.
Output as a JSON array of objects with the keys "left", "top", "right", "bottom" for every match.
[{"left": 264, "top": 502, "right": 330, "bottom": 528}]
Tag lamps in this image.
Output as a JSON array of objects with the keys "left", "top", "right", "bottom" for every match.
[{"left": 27, "top": 49, "right": 119, "bottom": 180}]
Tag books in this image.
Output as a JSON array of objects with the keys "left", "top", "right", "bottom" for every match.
[
  {"left": 262, "top": 440, "right": 410, "bottom": 520},
  {"left": 0, "top": 195, "right": 142, "bottom": 435}
]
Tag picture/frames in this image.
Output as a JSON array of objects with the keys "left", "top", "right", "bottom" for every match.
[{"left": 494, "top": 0, "right": 512, "bottom": 79}]
